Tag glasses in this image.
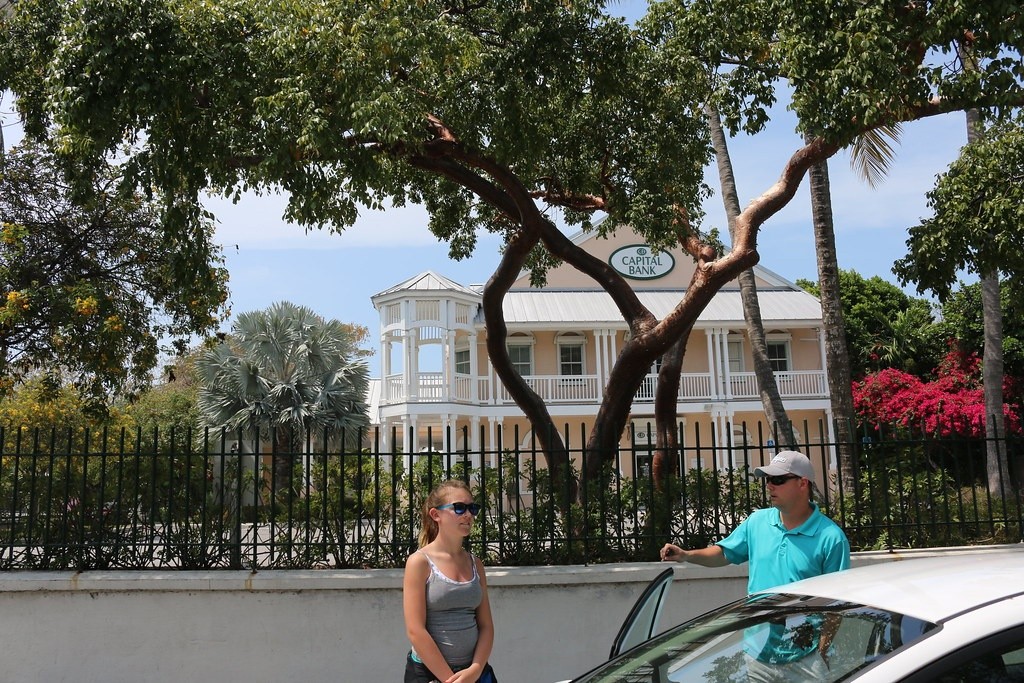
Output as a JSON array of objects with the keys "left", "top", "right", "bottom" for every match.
[
  {"left": 435, "top": 502, "right": 481, "bottom": 516},
  {"left": 766, "top": 475, "right": 799, "bottom": 485}
]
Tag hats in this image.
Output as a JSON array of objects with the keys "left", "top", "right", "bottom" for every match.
[{"left": 754, "top": 451, "right": 814, "bottom": 484}]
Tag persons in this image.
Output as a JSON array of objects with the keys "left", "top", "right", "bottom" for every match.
[
  {"left": 660, "top": 450, "right": 850, "bottom": 683},
  {"left": 403, "top": 480, "right": 497, "bottom": 683}
]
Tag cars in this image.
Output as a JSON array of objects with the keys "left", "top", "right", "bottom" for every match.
[{"left": 547, "top": 542, "right": 1024, "bottom": 683}]
